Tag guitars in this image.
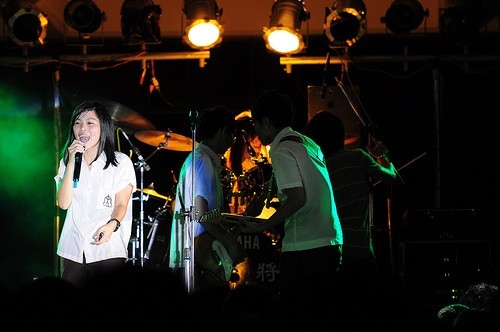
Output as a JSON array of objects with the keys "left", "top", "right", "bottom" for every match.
[
  {"left": 200, "top": 202, "right": 285, "bottom": 249},
  {"left": 194, "top": 197, "right": 265, "bottom": 289}
]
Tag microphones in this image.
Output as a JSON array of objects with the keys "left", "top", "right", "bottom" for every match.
[
  {"left": 120, "top": 129, "right": 150, "bottom": 171},
  {"left": 321, "top": 53, "right": 330, "bottom": 100},
  {"left": 73, "top": 152, "right": 82, "bottom": 187},
  {"left": 242, "top": 130, "right": 256, "bottom": 157}
]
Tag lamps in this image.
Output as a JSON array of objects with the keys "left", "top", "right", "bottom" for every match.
[
  {"left": 380, "top": 0, "right": 430, "bottom": 36},
  {"left": 263, "top": 0, "right": 311, "bottom": 58},
  {"left": 63, "top": 0, "right": 106, "bottom": 34},
  {"left": 0, "top": 0, "right": 51, "bottom": 48},
  {"left": 323, "top": 0, "right": 368, "bottom": 46},
  {"left": 122, "top": 0, "right": 161, "bottom": 44},
  {"left": 181, "top": 0, "right": 224, "bottom": 51}
]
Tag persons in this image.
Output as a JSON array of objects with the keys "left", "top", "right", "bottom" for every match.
[
  {"left": 244, "top": 94, "right": 344, "bottom": 270},
  {"left": 305, "top": 109, "right": 405, "bottom": 276},
  {"left": 52, "top": 102, "right": 135, "bottom": 275},
  {"left": 222, "top": 118, "right": 275, "bottom": 216},
  {"left": 167, "top": 106, "right": 235, "bottom": 273}
]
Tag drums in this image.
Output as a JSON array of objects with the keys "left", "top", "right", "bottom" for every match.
[
  {"left": 242, "top": 164, "right": 278, "bottom": 201},
  {"left": 221, "top": 167, "right": 237, "bottom": 203}
]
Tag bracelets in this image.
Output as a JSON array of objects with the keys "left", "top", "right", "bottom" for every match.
[{"left": 107, "top": 218, "right": 120, "bottom": 233}]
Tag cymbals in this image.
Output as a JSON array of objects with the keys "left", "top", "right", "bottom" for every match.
[
  {"left": 136, "top": 188, "right": 172, "bottom": 201},
  {"left": 135, "top": 130, "right": 198, "bottom": 151},
  {"left": 71, "top": 93, "right": 156, "bottom": 135},
  {"left": 372, "top": 152, "right": 427, "bottom": 188}
]
